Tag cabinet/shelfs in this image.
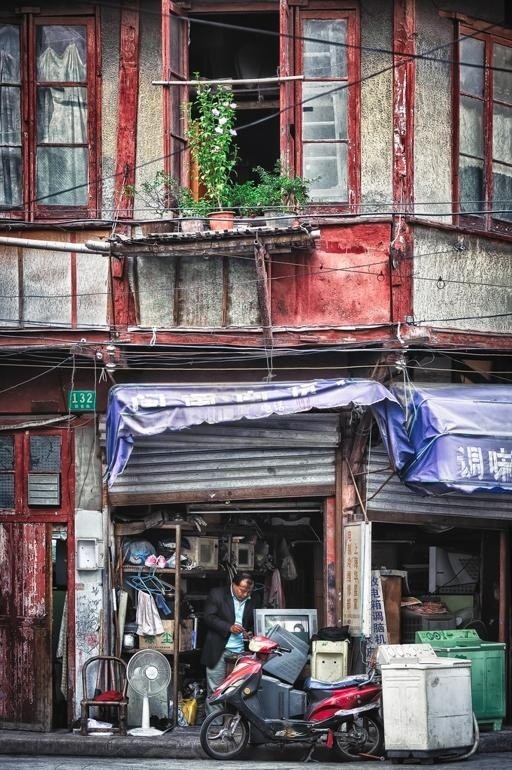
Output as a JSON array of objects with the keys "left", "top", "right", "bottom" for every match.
[{"left": 115, "top": 522, "right": 278, "bottom": 727}]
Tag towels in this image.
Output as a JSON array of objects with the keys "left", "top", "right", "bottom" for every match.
[{"left": 135, "top": 590, "right": 165, "bottom": 636}]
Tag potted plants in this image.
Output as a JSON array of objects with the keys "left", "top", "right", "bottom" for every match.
[{"left": 122, "top": 70, "right": 311, "bottom": 237}]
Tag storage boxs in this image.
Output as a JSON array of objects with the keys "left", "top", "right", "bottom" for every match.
[{"left": 139, "top": 619, "right": 193, "bottom": 651}]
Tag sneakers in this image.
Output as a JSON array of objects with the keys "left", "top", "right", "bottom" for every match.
[{"left": 145, "top": 554, "right": 165, "bottom": 569}]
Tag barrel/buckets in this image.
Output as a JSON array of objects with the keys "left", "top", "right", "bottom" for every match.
[{"left": 182, "top": 697, "right": 196, "bottom": 725}]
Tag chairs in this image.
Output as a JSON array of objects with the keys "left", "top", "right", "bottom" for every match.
[{"left": 80, "top": 654, "right": 129, "bottom": 736}]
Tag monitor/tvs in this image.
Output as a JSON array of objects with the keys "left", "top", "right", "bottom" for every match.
[
  {"left": 249, "top": 675, "right": 306, "bottom": 744},
  {"left": 253, "top": 609, "right": 318, "bottom": 661}
]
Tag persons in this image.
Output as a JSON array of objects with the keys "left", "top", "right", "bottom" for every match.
[{"left": 199, "top": 572, "right": 258, "bottom": 743}]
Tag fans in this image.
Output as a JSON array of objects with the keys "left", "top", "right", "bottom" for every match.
[{"left": 127, "top": 649, "right": 171, "bottom": 736}]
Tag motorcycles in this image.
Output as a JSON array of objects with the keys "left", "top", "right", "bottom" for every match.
[{"left": 199, "top": 626, "right": 384, "bottom": 764}]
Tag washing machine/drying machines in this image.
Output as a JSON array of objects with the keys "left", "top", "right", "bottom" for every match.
[
  {"left": 377, "top": 642, "right": 481, "bottom": 765},
  {"left": 416, "top": 627, "right": 508, "bottom": 730}
]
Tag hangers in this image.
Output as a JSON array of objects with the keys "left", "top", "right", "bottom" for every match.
[{"left": 125, "top": 567, "right": 176, "bottom": 597}]
